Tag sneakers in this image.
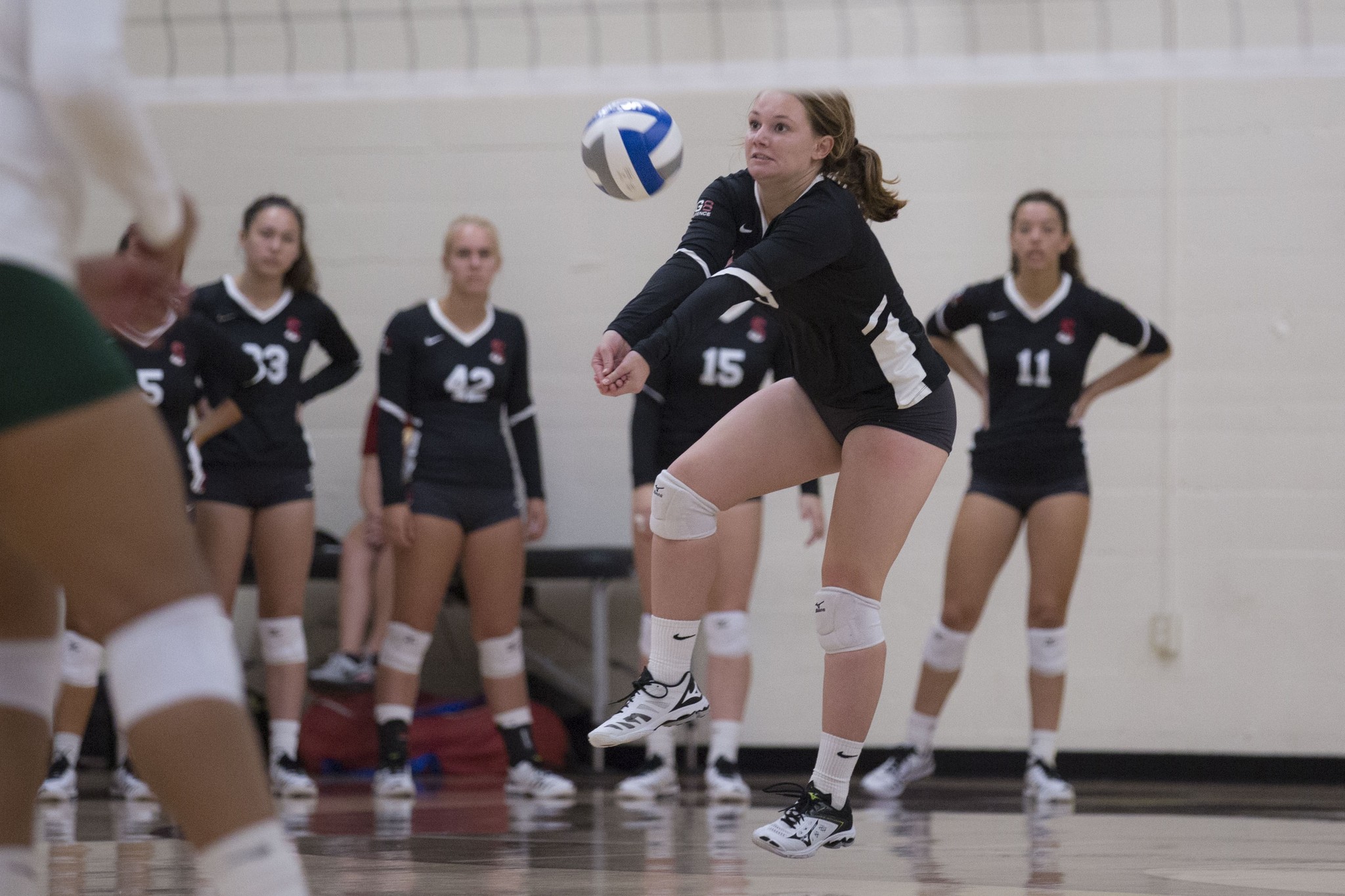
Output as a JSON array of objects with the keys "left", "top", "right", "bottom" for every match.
[
  {"left": 702, "top": 755, "right": 752, "bottom": 802},
  {"left": 614, "top": 755, "right": 681, "bottom": 799},
  {"left": 267, "top": 753, "right": 319, "bottom": 799},
  {"left": 102, "top": 765, "right": 158, "bottom": 802},
  {"left": 36, "top": 750, "right": 79, "bottom": 802},
  {"left": 860, "top": 745, "right": 935, "bottom": 798},
  {"left": 750, "top": 781, "right": 857, "bottom": 858},
  {"left": 369, "top": 762, "right": 417, "bottom": 797},
  {"left": 501, "top": 756, "right": 578, "bottom": 799},
  {"left": 587, "top": 665, "right": 711, "bottom": 746},
  {"left": 1021, "top": 756, "right": 1076, "bottom": 801}
]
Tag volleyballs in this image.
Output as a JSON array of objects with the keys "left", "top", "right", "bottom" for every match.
[{"left": 580, "top": 97, "right": 683, "bottom": 201}]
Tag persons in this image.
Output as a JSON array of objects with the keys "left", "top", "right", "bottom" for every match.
[
  {"left": 857, "top": 189, "right": 1171, "bottom": 803},
  {"left": 2, "top": 0, "right": 578, "bottom": 894},
  {"left": 585, "top": 91, "right": 958, "bottom": 860},
  {"left": 616, "top": 250, "right": 825, "bottom": 804}
]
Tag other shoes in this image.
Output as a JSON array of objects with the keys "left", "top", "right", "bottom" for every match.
[{"left": 308, "top": 651, "right": 376, "bottom": 686}]
